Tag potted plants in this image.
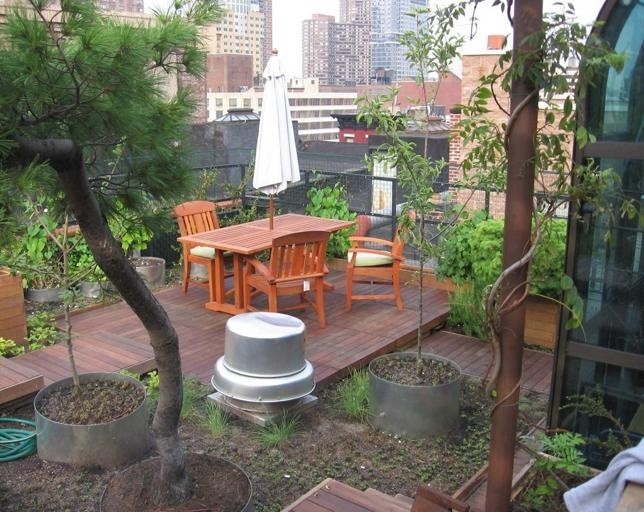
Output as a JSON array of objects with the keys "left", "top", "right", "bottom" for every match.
[{"left": 353, "top": 0, "right": 644, "bottom": 439}]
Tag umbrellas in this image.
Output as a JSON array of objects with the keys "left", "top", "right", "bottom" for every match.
[{"left": 253, "top": 49, "right": 301, "bottom": 230}]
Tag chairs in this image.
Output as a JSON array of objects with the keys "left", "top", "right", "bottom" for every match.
[{"left": 173, "top": 199, "right": 416, "bottom": 330}]
